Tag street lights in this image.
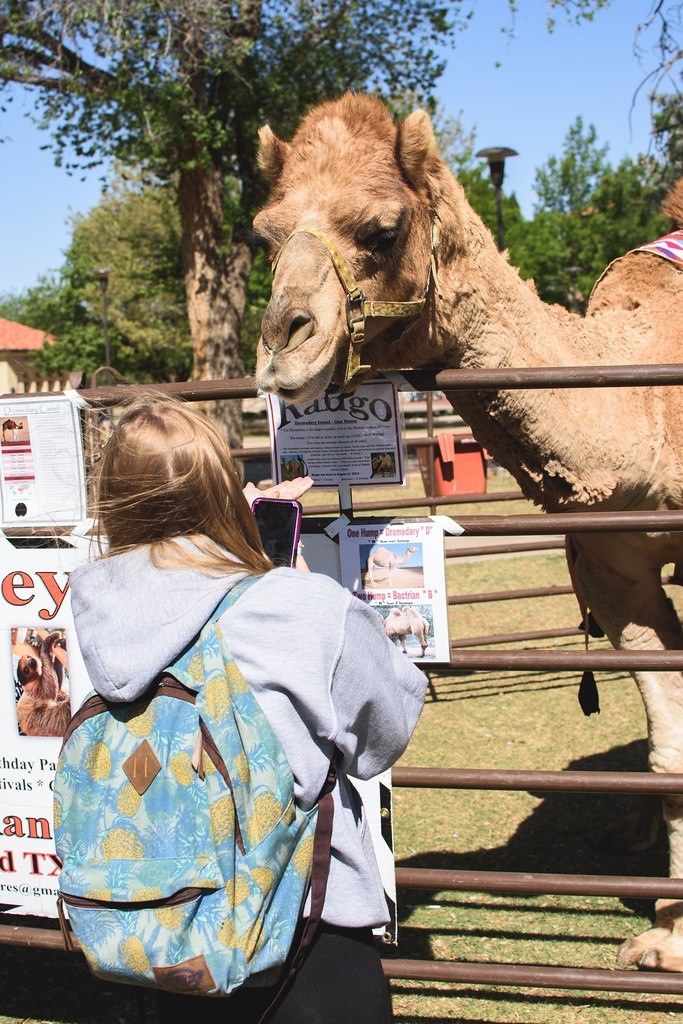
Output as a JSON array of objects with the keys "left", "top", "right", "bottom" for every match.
[
  {"left": 94, "top": 267, "right": 112, "bottom": 390},
  {"left": 478, "top": 146, "right": 519, "bottom": 258}
]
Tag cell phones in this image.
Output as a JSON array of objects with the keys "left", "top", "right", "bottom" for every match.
[{"left": 250, "top": 498, "right": 303, "bottom": 569}]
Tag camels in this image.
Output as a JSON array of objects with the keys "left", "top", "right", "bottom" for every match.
[
  {"left": 382, "top": 607, "right": 430, "bottom": 659},
  {"left": 281, "top": 457, "right": 302, "bottom": 480},
  {"left": 366, "top": 546, "right": 419, "bottom": 588},
  {"left": 371, "top": 456, "right": 395, "bottom": 477},
  {"left": 247, "top": 90, "right": 683, "bottom": 974}
]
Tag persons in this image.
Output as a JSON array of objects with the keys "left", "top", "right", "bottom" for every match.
[{"left": 75, "top": 392, "right": 432, "bottom": 1023}]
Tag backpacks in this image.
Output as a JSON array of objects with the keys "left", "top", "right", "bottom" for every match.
[{"left": 52, "top": 573, "right": 343, "bottom": 998}]
